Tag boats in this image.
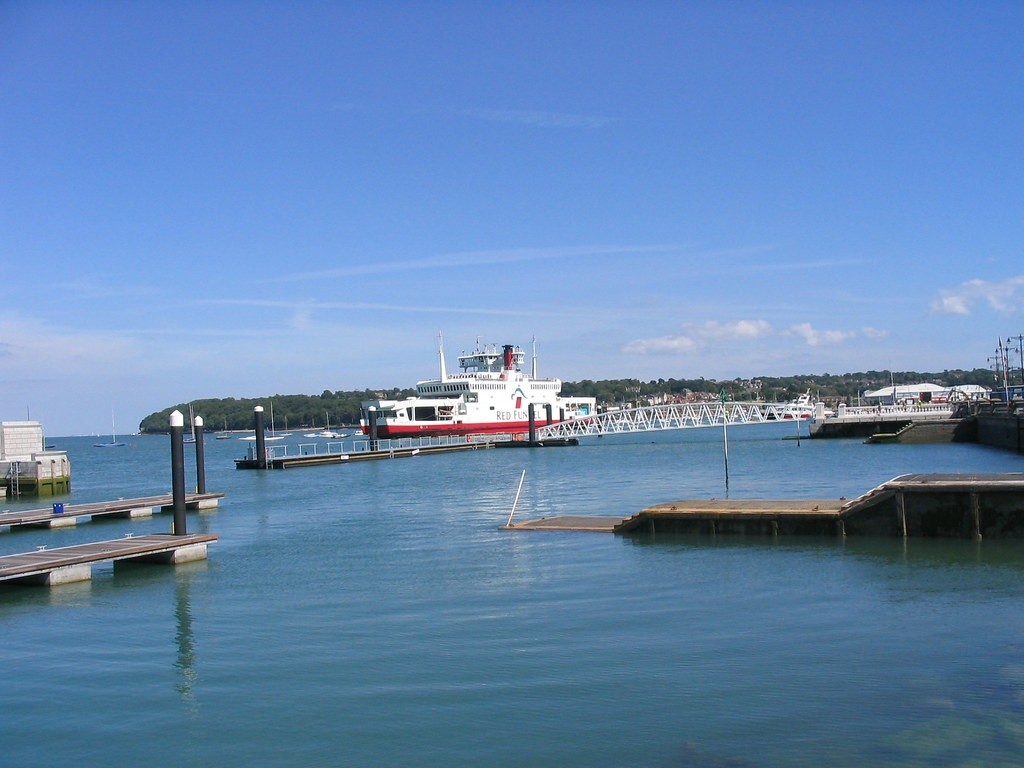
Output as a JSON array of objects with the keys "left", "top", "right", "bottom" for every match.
[
  {"left": 753, "top": 388, "right": 827, "bottom": 422},
  {"left": 359, "top": 328, "right": 600, "bottom": 435}
]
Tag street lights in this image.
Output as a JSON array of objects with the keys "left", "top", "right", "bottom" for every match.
[{"left": 987, "top": 334, "right": 1024, "bottom": 407}]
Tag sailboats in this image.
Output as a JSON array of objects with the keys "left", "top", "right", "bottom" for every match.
[
  {"left": 182, "top": 402, "right": 198, "bottom": 442},
  {"left": 93, "top": 409, "right": 127, "bottom": 448},
  {"left": 214, "top": 398, "right": 364, "bottom": 442}
]
